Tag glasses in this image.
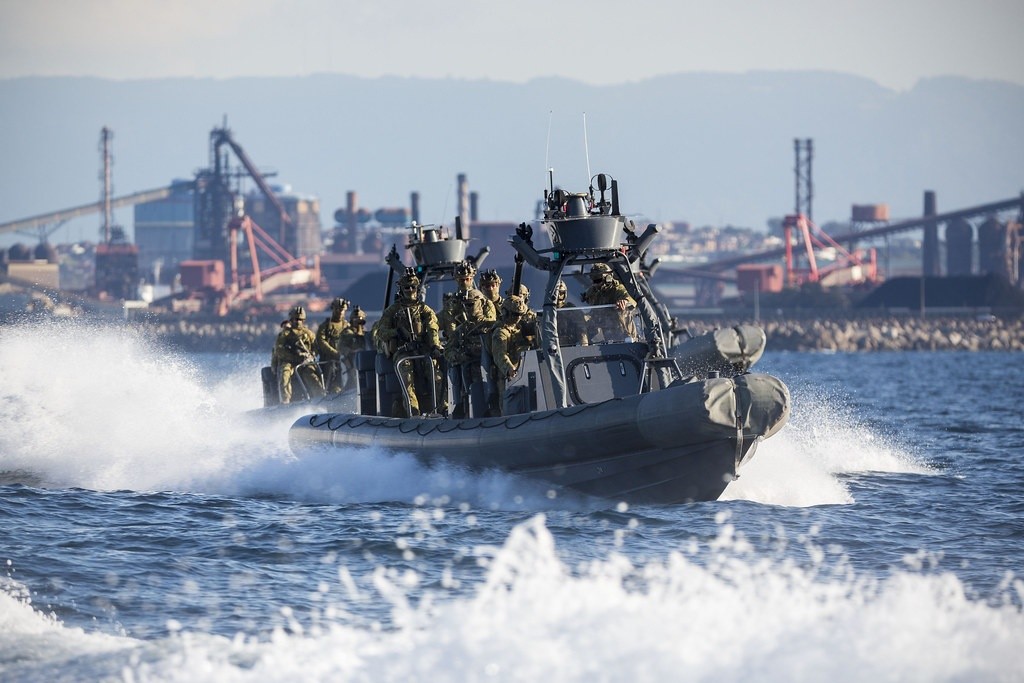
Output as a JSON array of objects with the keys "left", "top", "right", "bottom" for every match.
[
  {"left": 485, "top": 283, "right": 497, "bottom": 288},
  {"left": 404, "top": 285, "right": 417, "bottom": 289},
  {"left": 456, "top": 278, "right": 473, "bottom": 282}
]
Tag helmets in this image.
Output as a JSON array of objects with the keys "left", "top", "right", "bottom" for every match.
[
  {"left": 479, "top": 268, "right": 500, "bottom": 282},
  {"left": 590, "top": 263, "right": 613, "bottom": 280},
  {"left": 559, "top": 280, "right": 567, "bottom": 291},
  {"left": 331, "top": 299, "right": 350, "bottom": 309},
  {"left": 507, "top": 284, "right": 529, "bottom": 297},
  {"left": 502, "top": 296, "right": 524, "bottom": 313},
  {"left": 396, "top": 266, "right": 419, "bottom": 285},
  {"left": 350, "top": 306, "right": 366, "bottom": 318},
  {"left": 456, "top": 262, "right": 477, "bottom": 276},
  {"left": 289, "top": 306, "right": 306, "bottom": 319}
]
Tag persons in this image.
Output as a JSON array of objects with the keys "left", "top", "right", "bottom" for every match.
[
  {"left": 378, "top": 267, "right": 448, "bottom": 416},
  {"left": 557, "top": 279, "right": 589, "bottom": 347},
  {"left": 272, "top": 259, "right": 538, "bottom": 418},
  {"left": 580, "top": 262, "right": 638, "bottom": 343}
]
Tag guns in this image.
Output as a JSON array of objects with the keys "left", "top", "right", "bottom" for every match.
[
  {"left": 456, "top": 312, "right": 483, "bottom": 352},
  {"left": 393, "top": 306, "right": 422, "bottom": 376}
]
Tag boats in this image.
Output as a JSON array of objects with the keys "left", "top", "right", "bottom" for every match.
[{"left": 236, "top": 108, "right": 794, "bottom": 504}]
